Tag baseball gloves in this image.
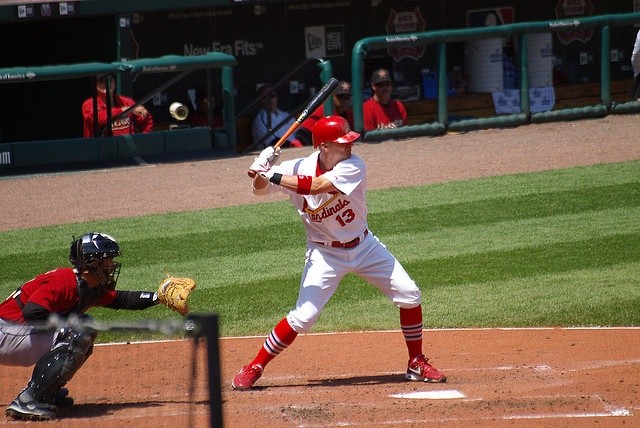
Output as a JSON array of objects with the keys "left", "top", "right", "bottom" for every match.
[{"left": 157, "top": 268, "right": 196, "bottom": 316}]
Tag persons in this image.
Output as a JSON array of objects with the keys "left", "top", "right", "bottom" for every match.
[
  {"left": 290, "top": 80, "right": 353, "bottom": 147},
  {"left": 251, "top": 85, "right": 301, "bottom": 153},
  {"left": 0, "top": 232, "right": 161, "bottom": 420},
  {"left": 627, "top": 27, "right": 640, "bottom": 101},
  {"left": 229, "top": 114, "right": 448, "bottom": 390},
  {"left": 362, "top": 68, "right": 408, "bottom": 131},
  {"left": 81, "top": 71, "right": 154, "bottom": 138}
]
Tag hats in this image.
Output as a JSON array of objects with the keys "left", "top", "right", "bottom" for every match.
[
  {"left": 371, "top": 70, "right": 391, "bottom": 86},
  {"left": 335, "top": 81, "right": 354, "bottom": 98},
  {"left": 94, "top": 72, "right": 116, "bottom": 81}
]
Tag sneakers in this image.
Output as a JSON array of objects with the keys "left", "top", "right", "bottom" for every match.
[
  {"left": 231, "top": 364, "right": 263, "bottom": 389},
  {"left": 5, "top": 392, "right": 57, "bottom": 421},
  {"left": 406, "top": 354, "right": 447, "bottom": 382}
]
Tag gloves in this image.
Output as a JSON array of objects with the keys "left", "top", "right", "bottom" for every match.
[
  {"left": 258, "top": 146, "right": 276, "bottom": 162},
  {"left": 248, "top": 159, "right": 274, "bottom": 179}
]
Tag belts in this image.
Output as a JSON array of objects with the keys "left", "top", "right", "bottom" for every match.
[{"left": 314, "top": 229, "right": 369, "bottom": 247}]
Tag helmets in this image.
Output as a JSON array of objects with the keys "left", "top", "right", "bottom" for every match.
[
  {"left": 313, "top": 115, "right": 361, "bottom": 148},
  {"left": 69, "top": 232, "right": 120, "bottom": 290}
]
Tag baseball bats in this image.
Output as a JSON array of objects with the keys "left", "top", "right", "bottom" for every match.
[{"left": 248, "top": 78, "right": 340, "bottom": 178}]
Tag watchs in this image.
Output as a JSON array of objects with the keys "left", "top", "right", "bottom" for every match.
[{"left": 152, "top": 293, "right": 157, "bottom": 300}]
